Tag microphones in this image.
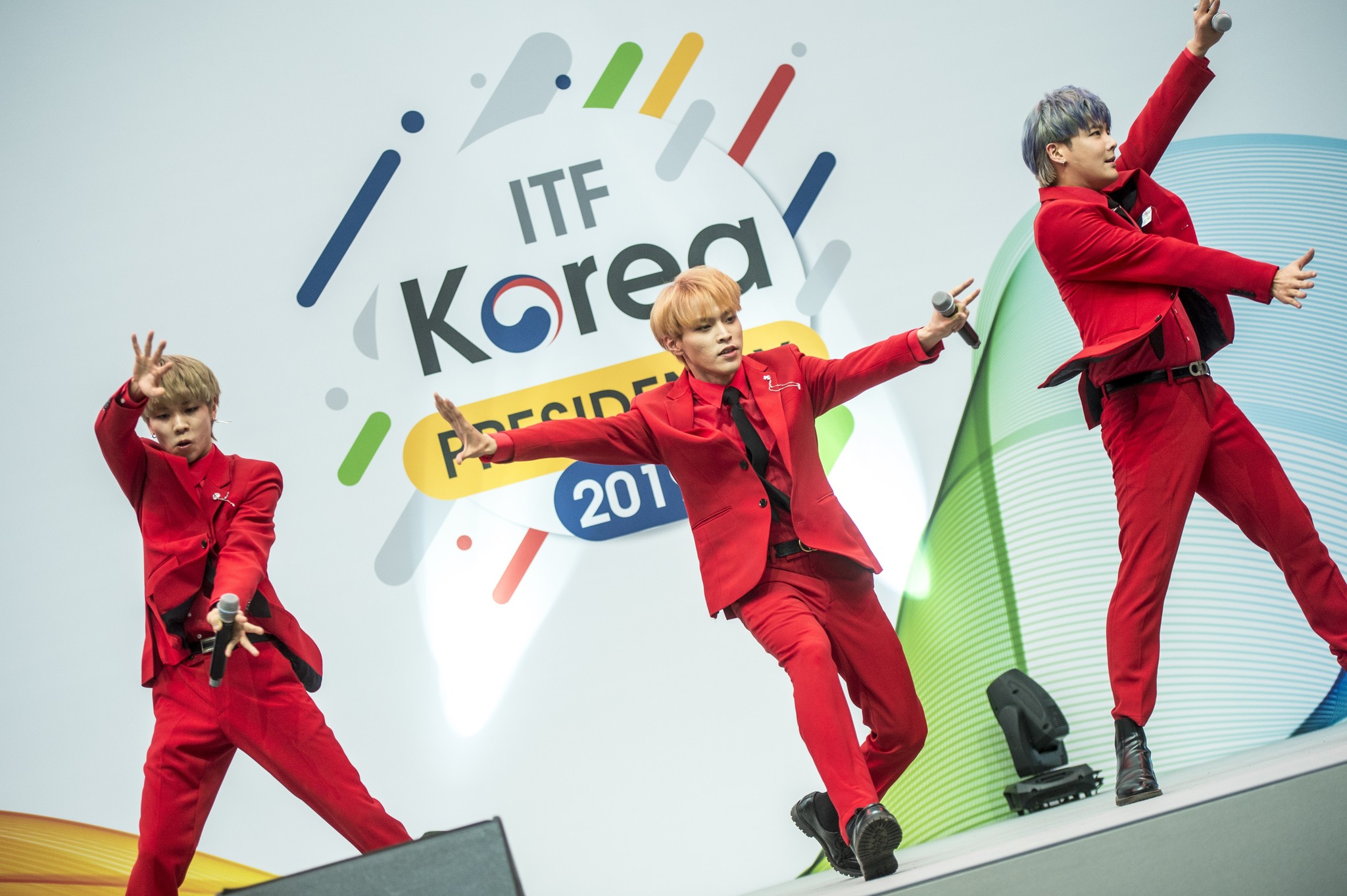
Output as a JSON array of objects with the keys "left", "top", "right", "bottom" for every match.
[
  {"left": 1193, "top": 3, "right": 1232, "bottom": 32},
  {"left": 931, "top": 290, "right": 982, "bottom": 349},
  {"left": 209, "top": 593, "right": 239, "bottom": 687}
]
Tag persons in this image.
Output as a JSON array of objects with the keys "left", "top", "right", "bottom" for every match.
[
  {"left": 97, "top": 333, "right": 411, "bottom": 896},
  {"left": 1022, "top": 0, "right": 1347, "bottom": 805},
  {"left": 434, "top": 267, "right": 982, "bottom": 882}
]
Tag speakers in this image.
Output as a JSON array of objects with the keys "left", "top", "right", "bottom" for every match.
[{"left": 218, "top": 815, "right": 525, "bottom": 896}]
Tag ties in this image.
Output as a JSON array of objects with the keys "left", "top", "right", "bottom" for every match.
[
  {"left": 722, "top": 384, "right": 791, "bottom": 521},
  {"left": 1105, "top": 195, "right": 1166, "bottom": 360}
]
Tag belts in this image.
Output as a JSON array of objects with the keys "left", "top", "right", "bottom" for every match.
[
  {"left": 189, "top": 628, "right": 274, "bottom": 653},
  {"left": 768, "top": 536, "right": 818, "bottom": 558},
  {"left": 1098, "top": 360, "right": 1209, "bottom": 398}
]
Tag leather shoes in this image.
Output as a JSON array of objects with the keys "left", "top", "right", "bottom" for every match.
[
  {"left": 845, "top": 802, "right": 902, "bottom": 881},
  {"left": 791, "top": 792, "right": 861, "bottom": 877},
  {"left": 1113, "top": 715, "right": 1162, "bottom": 804}
]
